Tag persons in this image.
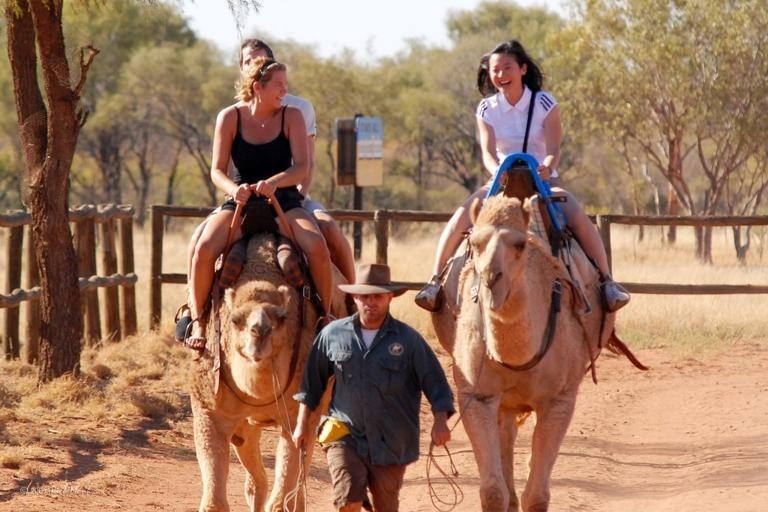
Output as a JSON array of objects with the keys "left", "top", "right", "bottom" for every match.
[
  {"left": 176, "top": 38, "right": 355, "bottom": 340},
  {"left": 292, "top": 264, "right": 457, "bottom": 512},
  {"left": 414, "top": 40, "right": 630, "bottom": 311},
  {"left": 184, "top": 55, "right": 335, "bottom": 349}
]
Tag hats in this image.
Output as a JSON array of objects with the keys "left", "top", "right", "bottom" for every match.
[{"left": 337, "top": 263, "right": 408, "bottom": 295}]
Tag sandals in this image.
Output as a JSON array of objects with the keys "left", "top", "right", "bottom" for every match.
[{"left": 187, "top": 321, "right": 208, "bottom": 350}]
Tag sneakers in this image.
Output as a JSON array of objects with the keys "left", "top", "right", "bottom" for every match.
[
  {"left": 605, "top": 283, "right": 631, "bottom": 311},
  {"left": 417, "top": 278, "right": 442, "bottom": 309},
  {"left": 175, "top": 316, "right": 193, "bottom": 341}
]
